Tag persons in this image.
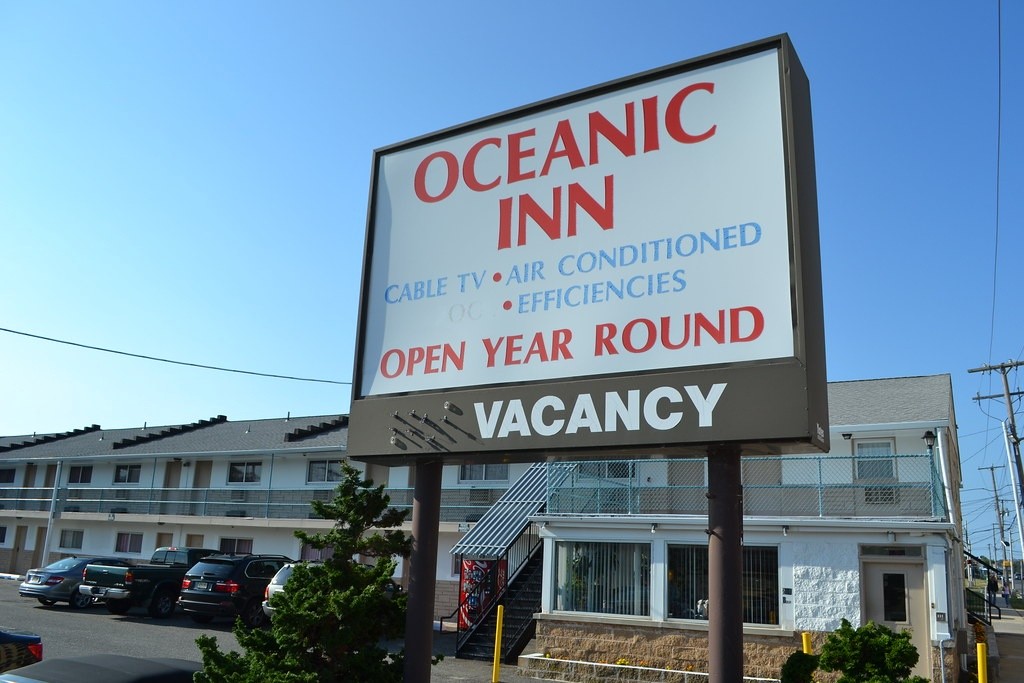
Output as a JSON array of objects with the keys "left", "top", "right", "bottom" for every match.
[
  {"left": 986, "top": 575, "right": 999, "bottom": 607},
  {"left": 1002, "top": 582, "right": 1011, "bottom": 607}
]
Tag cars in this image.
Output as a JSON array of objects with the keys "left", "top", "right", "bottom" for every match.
[
  {"left": 18, "top": 555, "right": 133, "bottom": 609},
  {"left": 0, "top": 653, "right": 205, "bottom": 683},
  {"left": 1013, "top": 572, "right": 1021, "bottom": 580},
  {"left": 0, "top": 625, "right": 44, "bottom": 674}
]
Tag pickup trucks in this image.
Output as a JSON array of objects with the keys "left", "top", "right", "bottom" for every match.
[{"left": 78, "top": 545, "right": 228, "bottom": 619}]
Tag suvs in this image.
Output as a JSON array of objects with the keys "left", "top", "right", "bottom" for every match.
[
  {"left": 176, "top": 551, "right": 295, "bottom": 626},
  {"left": 263, "top": 560, "right": 403, "bottom": 619}
]
